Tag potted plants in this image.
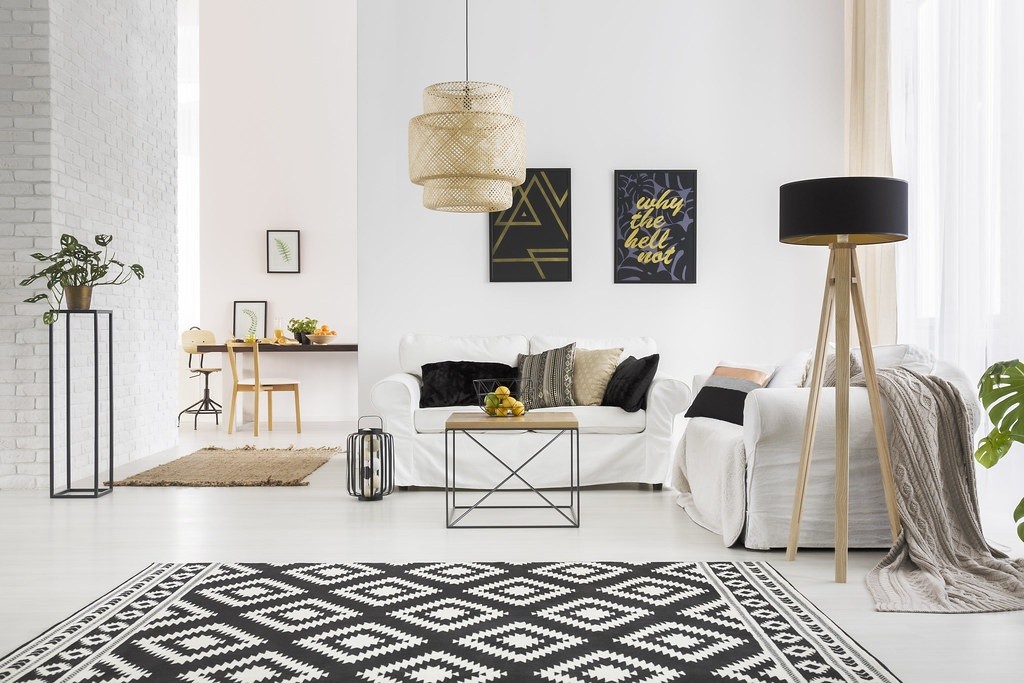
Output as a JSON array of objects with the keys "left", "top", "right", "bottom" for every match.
[{"left": 18, "top": 233, "right": 144, "bottom": 324}]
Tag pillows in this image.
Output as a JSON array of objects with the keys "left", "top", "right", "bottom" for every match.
[
  {"left": 517, "top": 342, "right": 575, "bottom": 410},
  {"left": 573, "top": 347, "right": 624, "bottom": 406},
  {"left": 684, "top": 359, "right": 780, "bottom": 426},
  {"left": 600, "top": 353, "right": 659, "bottom": 413},
  {"left": 420, "top": 360, "right": 519, "bottom": 408}
]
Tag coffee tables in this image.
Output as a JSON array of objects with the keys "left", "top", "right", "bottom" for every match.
[{"left": 444, "top": 411, "right": 579, "bottom": 529}]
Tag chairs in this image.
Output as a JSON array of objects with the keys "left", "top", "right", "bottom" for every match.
[
  {"left": 227, "top": 341, "right": 302, "bottom": 436},
  {"left": 178, "top": 327, "right": 222, "bottom": 430}
]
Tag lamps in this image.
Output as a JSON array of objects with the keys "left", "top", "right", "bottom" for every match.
[
  {"left": 407, "top": 0, "right": 527, "bottom": 214},
  {"left": 779, "top": 177, "right": 909, "bottom": 585}
]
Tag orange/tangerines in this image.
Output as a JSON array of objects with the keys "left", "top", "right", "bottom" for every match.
[
  {"left": 314, "top": 325, "right": 337, "bottom": 335},
  {"left": 278, "top": 338, "right": 286, "bottom": 344}
]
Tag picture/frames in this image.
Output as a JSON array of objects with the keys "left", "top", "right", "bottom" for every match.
[
  {"left": 266, "top": 230, "right": 300, "bottom": 273},
  {"left": 233, "top": 300, "right": 267, "bottom": 342}
]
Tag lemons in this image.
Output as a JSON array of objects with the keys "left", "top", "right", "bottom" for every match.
[{"left": 484, "top": 386, "right": 526, "bottom": 417}]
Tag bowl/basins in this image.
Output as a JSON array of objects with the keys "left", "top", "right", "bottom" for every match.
[{"left": 306, "top": 335, "right": 336, "bottom": 345}]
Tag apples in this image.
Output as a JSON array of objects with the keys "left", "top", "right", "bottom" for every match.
[{"left": 484, "top": 394, "right": 499, "bottom": 407}]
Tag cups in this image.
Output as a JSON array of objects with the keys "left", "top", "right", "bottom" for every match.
[
  {"left": 274, "top": 331, "right": 284, "bottom": 343},
  {"left": 246, "top": 334, "right": 256, "bottom": 343}
]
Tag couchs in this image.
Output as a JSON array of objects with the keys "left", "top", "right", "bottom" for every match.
[
  {"left": 370, "top": 332, "right": 692, "bottom": 491},
  {"left": 663, "top": 339, "right": 983, "bottom": 550}
]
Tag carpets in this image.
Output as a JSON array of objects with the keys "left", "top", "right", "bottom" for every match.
[
  {"left": 0, "top": 562, "right": 903, "bottom": 683},
  {"left": 103, "top": 444, "right": 348, "bottom": 486}
]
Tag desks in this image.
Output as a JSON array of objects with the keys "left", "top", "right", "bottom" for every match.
[
  {"left": 49, "top": 309, "right": 114, "bottom": 498},
  {"left": 196, "top": 344, "right": 356, "bottom": 352}
]
